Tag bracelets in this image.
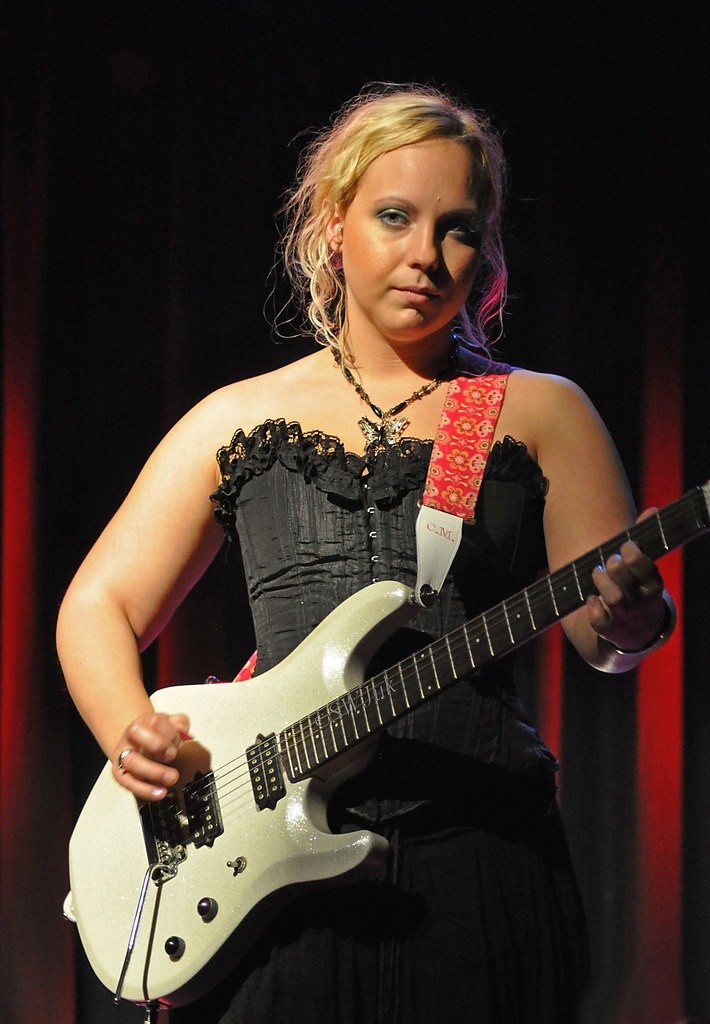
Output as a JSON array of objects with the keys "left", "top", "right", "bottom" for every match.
[{"left": 597, "top": 598, "right": 671, "bottom": 655}]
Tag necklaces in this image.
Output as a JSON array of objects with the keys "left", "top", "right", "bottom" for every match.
[{"left": 329, "top": 338, "right": 459, "bottom": 443}]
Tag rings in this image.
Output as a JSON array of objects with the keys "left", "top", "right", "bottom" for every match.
[{"left": 118, "top": 749, "right": 133, "bottom": 767}]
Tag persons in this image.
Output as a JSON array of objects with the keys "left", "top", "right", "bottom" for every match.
[{"left": 53, "top": 90, "right": 675, "bottom": 1022}]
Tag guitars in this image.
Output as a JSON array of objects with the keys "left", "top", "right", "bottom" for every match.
[{"left": 67, "top": 475, "right": 710, "bottom": 1007}]
